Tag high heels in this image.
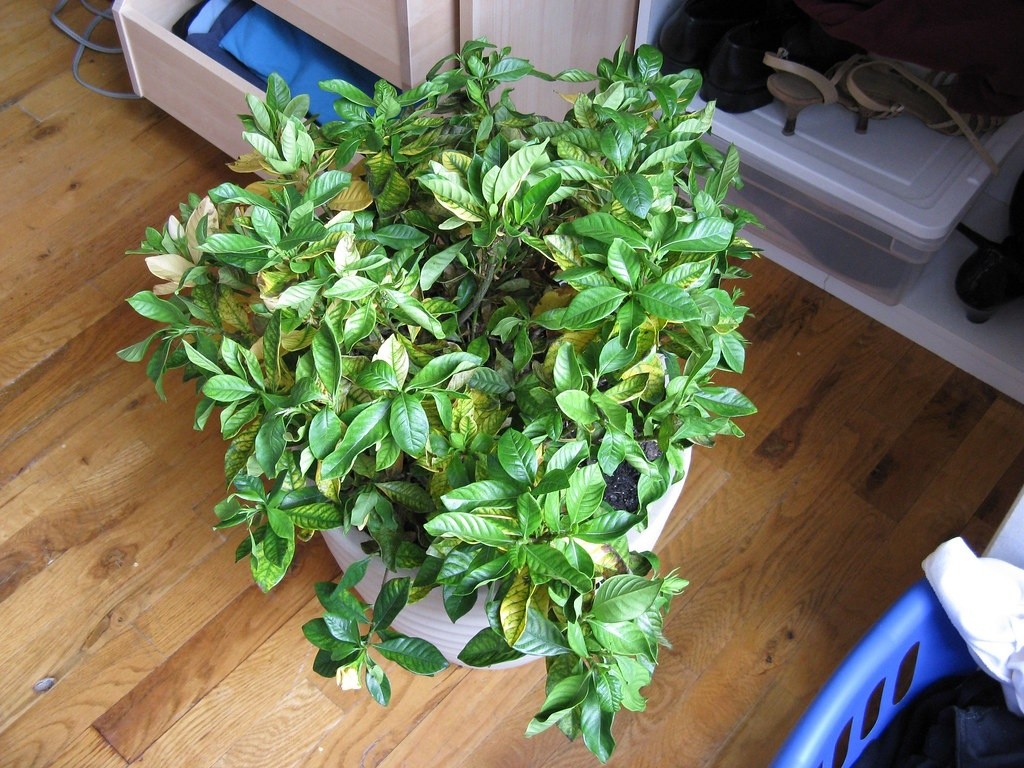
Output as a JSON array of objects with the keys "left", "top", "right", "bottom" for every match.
[
  {"left": 846, "top": 59, "right": 1008, "bottom": 178},
  {"left": 954, "top": 239, "right": 1024, "bottom": 324},
  {"left": 763, "top": 47, "right": 902, "bottom": 136}
]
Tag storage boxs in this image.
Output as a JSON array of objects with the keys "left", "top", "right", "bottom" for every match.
[{"left": 646, "top": 75, "right": 1024, "bottom": 306}]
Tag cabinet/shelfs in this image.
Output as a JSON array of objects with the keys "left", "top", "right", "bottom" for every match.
[{"left": 110, "top": 0, "right": 640, "bottom": 289}]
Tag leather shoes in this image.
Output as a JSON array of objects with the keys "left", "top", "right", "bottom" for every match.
[{"left": 657, "top": 2, "right": 859, "bottom": 114}]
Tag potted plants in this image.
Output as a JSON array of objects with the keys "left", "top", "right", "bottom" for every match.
[{"left": 114, "top": 38, "right": 763, "bottom": 765}]
